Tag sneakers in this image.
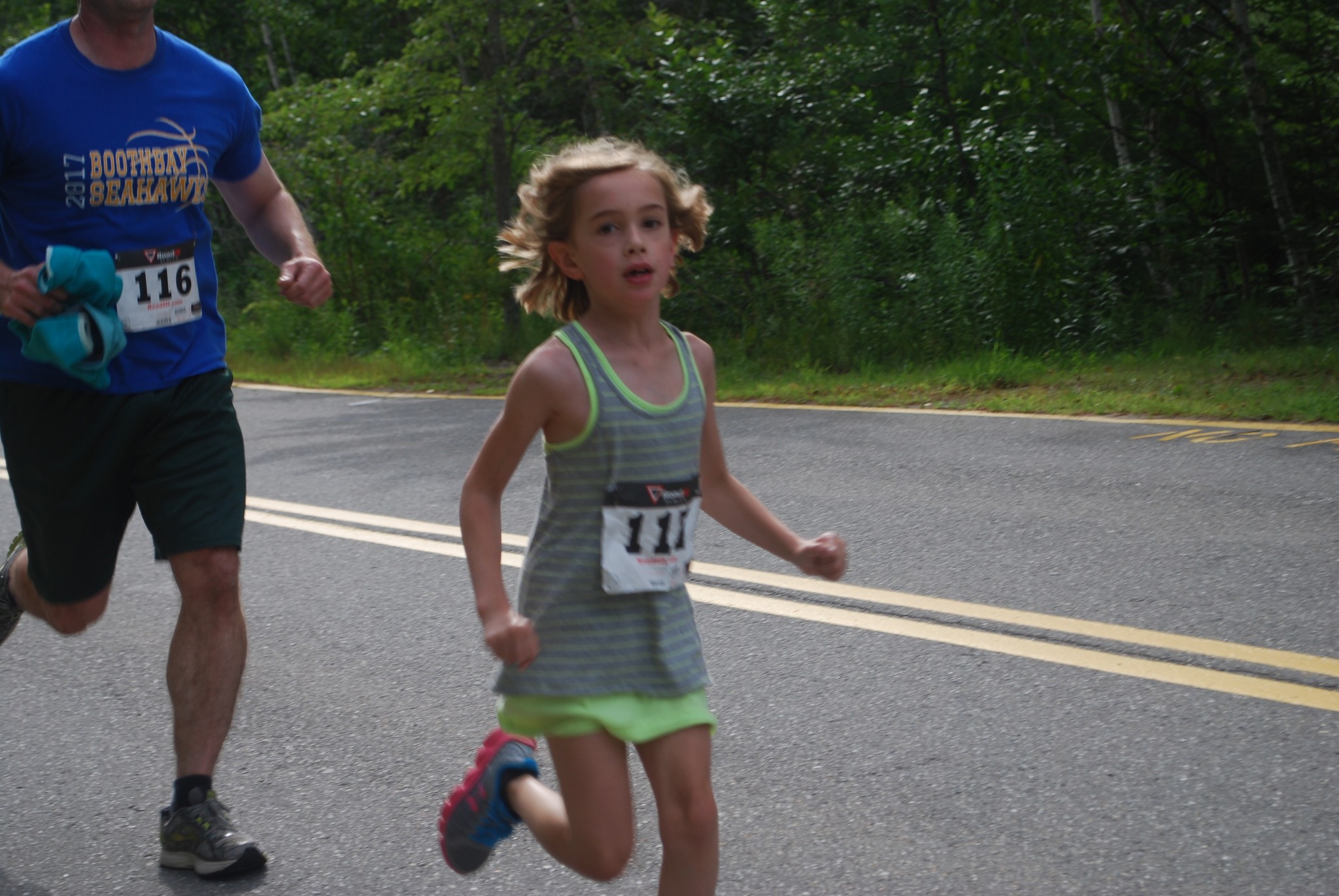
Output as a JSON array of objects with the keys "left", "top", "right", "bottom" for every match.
[
  {"left": 159, "top": 790, "right": 267, "bottom": 880},
  {"left": 0, "top": 530, "right": 27, "bottom": 646},
  {"left": 440, "top": 727, "right": 538, "bottom": 875}
]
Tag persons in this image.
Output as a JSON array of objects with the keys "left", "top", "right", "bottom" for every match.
[
  {"left": 0, "top": 0, "right": 334, "bottom": 883},
  {"left": 438, "top": 141, "right": 846, "bottom": 896}
]
